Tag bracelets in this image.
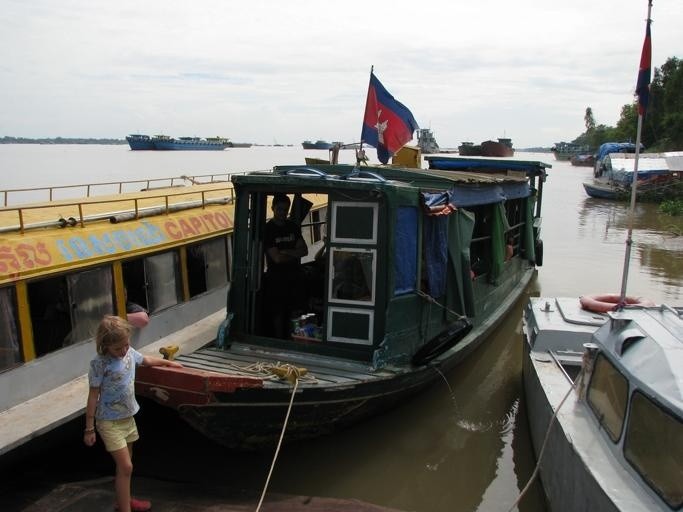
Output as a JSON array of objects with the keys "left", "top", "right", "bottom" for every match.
[{"left": 85, "top": 425, "right": 95, "bottom": 434}]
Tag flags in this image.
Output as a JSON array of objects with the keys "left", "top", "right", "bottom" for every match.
[
  {"left": 634, "top": 23, "right": 653, "bottom": 122},
  {"left": 362, "top": 73, "right": 420, "bottom": 164}
]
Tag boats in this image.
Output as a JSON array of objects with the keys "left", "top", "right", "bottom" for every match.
[
  {"left": 301, "top": 139, "right": 332, "bottom": 149},
  {"left": 456, "top": 138, "right": 514, "bottom": 156},
  {"left": 123, "top": 132, "right": 252, "bottom": 152},
  {"left": 570, "top": 142, "right": 681, "bottom": 201},
  {"left": 416, "top": 128, "right": 438, "bottom": 153},
  {"left": 519, "top": 295, "right": 680, "bottom": 511}
]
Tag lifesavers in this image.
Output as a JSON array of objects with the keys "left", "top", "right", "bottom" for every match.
[{"left": 580, "top": 294, "right": 655, "bottom": 312}]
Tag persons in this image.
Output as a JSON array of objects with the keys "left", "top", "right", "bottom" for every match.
[
  {"left": 104, "top": 286, "right": 149, "bottom": 328},
  {"left": 264, "top": 195, "right": 308, "bottom": 321},
  {"left": 316, "top": 235, "right": 370, "bottom": 299},
  {"left": 82, "top": 316, "right": 184, "bottom": 512}
]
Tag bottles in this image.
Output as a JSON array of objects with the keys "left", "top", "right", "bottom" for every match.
[{"left": 219, "top": 326, "right": 224, "bottom": 347}]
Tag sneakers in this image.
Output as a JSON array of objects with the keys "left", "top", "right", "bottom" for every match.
[{"left": 114, "top": 497, "right": 150, "bottom": 511}]
